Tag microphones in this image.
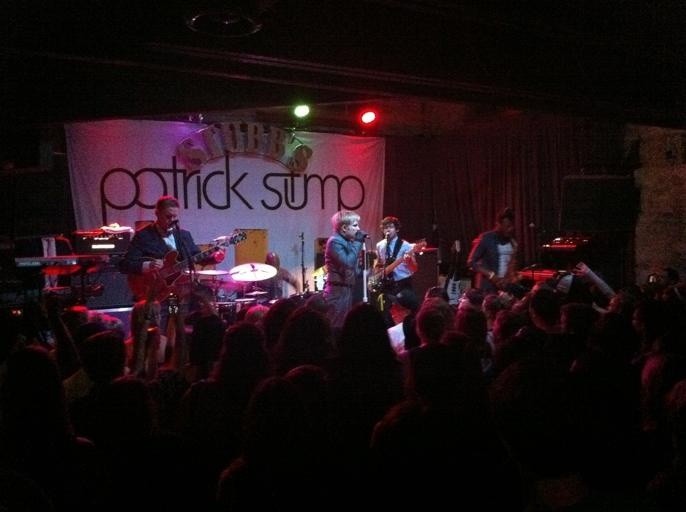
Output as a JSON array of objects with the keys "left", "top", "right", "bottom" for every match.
[
  {"left": 354, "top": 229, "right": 371, "bottom": 244},
  {"left": 383, "top": 230, "right": 392, "bottom": 242},
  {"left": 166, "top": 218, "right": 180, "bottom": 230}
]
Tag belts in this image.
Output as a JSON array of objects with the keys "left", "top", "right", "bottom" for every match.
[{"left": 326, "top": 281, "right": 352, "bottom": 288}]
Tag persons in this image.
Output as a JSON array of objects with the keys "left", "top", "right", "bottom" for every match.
[
  {"left": 466, "top": 206, "right": 519, "bottom": 290},
  {"left": 322, "top": 209, "right": 369, "bottom": 326},
  {"left": 376, "top": 216, "right": 418, "bottom": 300},
  {"left": 0, "top": 261, "right": 685, "bottom": 512},
  {"left": 247, "top": 252, "right": 301, "bottom": 297},
  {"left": 115, "top": 195, "right": 226, "bottom": 320}
]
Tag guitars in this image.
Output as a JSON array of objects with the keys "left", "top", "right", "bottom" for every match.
[
  {"left": 127, "top": 232, "right": 247, "bottom": 303},
  {"left": 367, "top": 240, "right": 428, "bottom": 295}
]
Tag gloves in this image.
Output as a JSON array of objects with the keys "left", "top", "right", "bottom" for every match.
[{"left": 355, "top": 230, "right": 369, "bottom": 242}]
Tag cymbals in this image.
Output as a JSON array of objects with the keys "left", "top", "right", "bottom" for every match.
[
  {"left": 184, "top": 269, "right": 229, "bottom": 275},
  {"left": 230, "top": 262, "right": 277, "bottom": 283}
]
